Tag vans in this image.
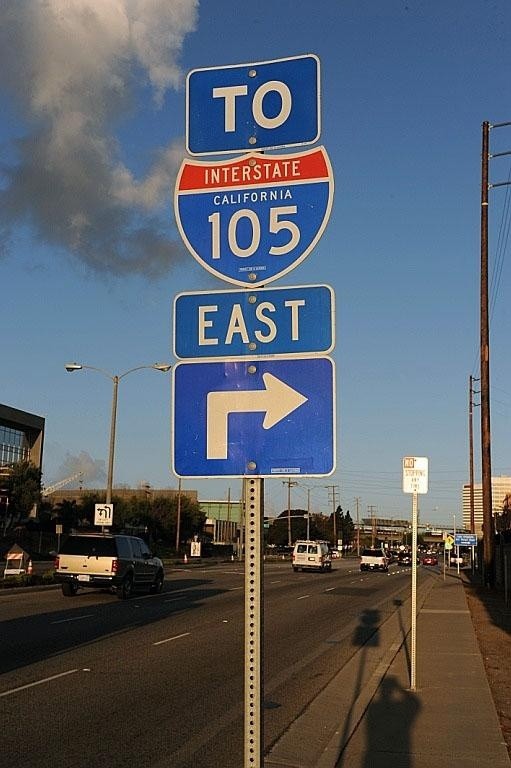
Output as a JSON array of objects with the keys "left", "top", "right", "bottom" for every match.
[{"left": 293, "top": 539, "right": 332, "bottom": 573}]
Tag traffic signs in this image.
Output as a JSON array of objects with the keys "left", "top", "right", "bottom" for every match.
[{"left": 94, "top": 503, "right": 113, "bottom": 526}]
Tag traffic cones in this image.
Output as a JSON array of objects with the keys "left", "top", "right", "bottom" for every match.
[
  {"left": 281, "top": 554, "right": 286, "bottom": 560},
  {"left": 27, "top": 559, "right": 34, "bottom": 574},
  {"left": 231, "top": 555, "right": 235, "bottom": 560},
  {"left": 183, "top": 553, "right": 188, "bottom": 563}
]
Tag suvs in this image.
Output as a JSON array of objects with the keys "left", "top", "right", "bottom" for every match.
[{"left": 54, "top": 533, "right": 166, "bottom": 598}]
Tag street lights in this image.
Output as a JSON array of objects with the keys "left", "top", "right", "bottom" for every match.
[
  {"left": 63, "top": 360, "right": 175, "bottom": 528},
  {"left": 452, "top": 513, "right": 459, "bottom": 554}
]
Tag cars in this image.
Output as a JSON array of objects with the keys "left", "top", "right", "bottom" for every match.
[
  {"left": 359, "top": 547, "right": 440, "bottom": 572},
  {"left": 329, "top": 547, "right": 340, "bottom": 557}
]
